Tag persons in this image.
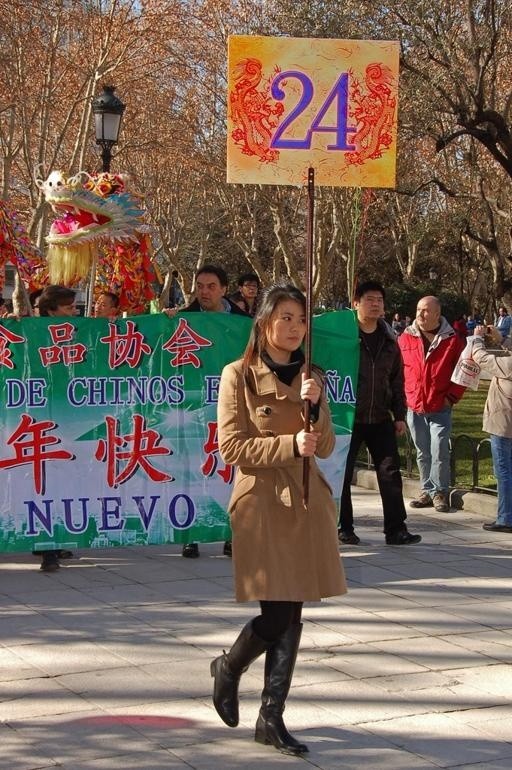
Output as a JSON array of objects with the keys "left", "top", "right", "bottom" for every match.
[
  {"left": 398, "top": 294, "right": 475, "bottom": 512},
  {"left": 234, "top": 274, "right": 262, "bottom": 317},
  {"left": 162, "top": 265, "right": 245, "bottom": 319},
  {"left": 472, "top": 316, "right": 512, "bottom": 532},
  {"left": 336, "top": 281, "right": 421, "bottom": 547},
  {"left": 385, "top": 307, "right": 512, "bottom": 336},
  {"left": 93, "top": 293, "right": 119, "bottom": 317},
  {"left": 1, "top": 300, "right": 21, "bottom": 319},
  {"left": 40, "top": 284, "right": 78, "bottom": 316},
  {"left": 30, "top": 288, "right": 45, "bottom": 319},
  {"left": 206, "top": 284, "right": 346, "bottom": 756}
]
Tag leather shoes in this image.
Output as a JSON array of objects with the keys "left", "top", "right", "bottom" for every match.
[
  {"left": 384, "top": 527, "right": 424, "bottom": 545},
  {"left": 337, "top": 527, "right": 361, "bottom": 546},
  {"left": 182, "top": 544, "right": 201, "bottom": 559},
  {"left": 480, "top": 521, "right": 512, "bottom": 533},
  {"left": 224, "top": 542, "right": 232, "bottom": 555}
]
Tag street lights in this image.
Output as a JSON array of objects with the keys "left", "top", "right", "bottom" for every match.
[
  {"left": 428, "top": 268, "right": 439, "bottom": 295},
  {"left": 88, "top": 82, "right": 128, "bottom": 173}
]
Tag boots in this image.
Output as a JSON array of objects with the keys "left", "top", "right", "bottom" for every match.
[
  {"left": 208, "top": 617, "right": 279, "bottom": 728},
  {"left": 252, "top": 619, "right": 314, "bottom": 762}
]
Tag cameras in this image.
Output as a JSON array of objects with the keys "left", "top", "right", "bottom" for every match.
[{"left": 482, "top": 327, "right": 490, "bottom": 334}]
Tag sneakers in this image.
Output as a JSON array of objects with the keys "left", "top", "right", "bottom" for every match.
[
  {"left": 31, "top": 547, "right": 74, "bottom": 559},
  {"left": 431, "top": 493, "right": 450, "bottom": 513},
  {"left": 40, "top": 549, "right": 60, "bottom": 572},
  {"left": 408, "top": 492, "right": 433, "bottom": 509}
]
